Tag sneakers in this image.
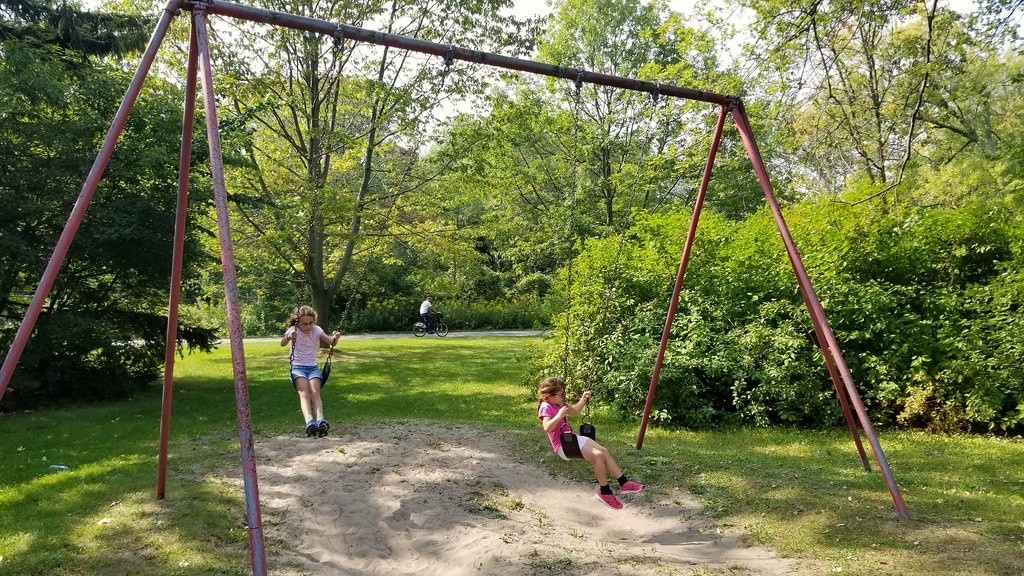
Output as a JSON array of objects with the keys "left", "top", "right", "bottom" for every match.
[
  {"left": 305, "top": 418, "right": 330, "bottom": 438},
  {"left": 596, "top": 488, "right": 624, "bottom": 510},
  {"left": 620, "top": 481, "right": 645, "bottom": 494}
]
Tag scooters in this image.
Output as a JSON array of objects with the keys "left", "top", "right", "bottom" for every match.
[{"left": 413, "top": 310, "right": 449, "bottom": 337}]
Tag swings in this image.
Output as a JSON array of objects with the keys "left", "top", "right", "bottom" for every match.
[
  {"left": 288, "top": 33, "right": 455, "bottom": 393},
  {"left": 559, "top": 79, "right": 660, "bottom": 460}
]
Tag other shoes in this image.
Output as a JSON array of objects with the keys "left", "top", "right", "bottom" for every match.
[{"left": 428, "top": 331, "right": 435, "bottom": 334}]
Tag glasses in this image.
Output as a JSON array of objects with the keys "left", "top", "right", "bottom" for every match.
[
  {"left": 554, "top": 392, "right": 566, "bottom": 398},
  {"left": 299, "top": 321, "right": 315, "bottom": 327}
]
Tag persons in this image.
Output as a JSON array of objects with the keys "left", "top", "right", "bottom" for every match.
[
  {"left": 280, "top": 305, "right": 341, "bottom": 438},
  {"left": 537, "top": 377, "right": 645, "bottom": 510},
  {"left": 420, "top": 297, "right": 438, "bottom": 334}
]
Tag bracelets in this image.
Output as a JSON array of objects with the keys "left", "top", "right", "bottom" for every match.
[{"left": 287, "top": 333, "right": 291, "bottom": 340}]
